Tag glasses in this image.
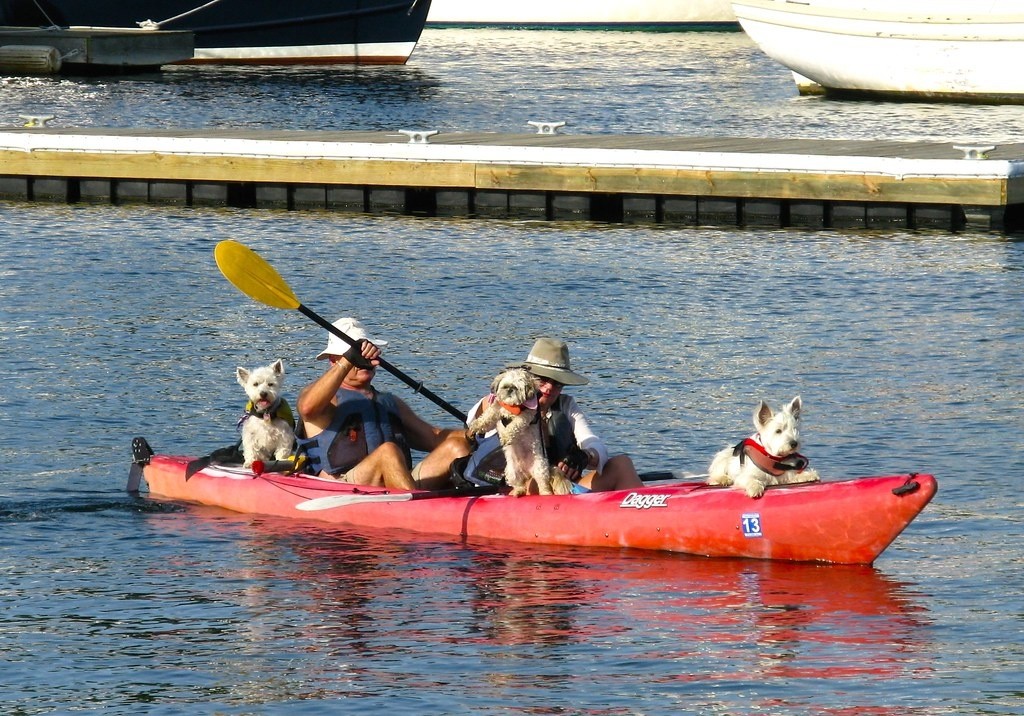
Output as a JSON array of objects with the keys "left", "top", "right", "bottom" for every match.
[{"left": 539, "top": 376, "right": 562, "bottom": 387}]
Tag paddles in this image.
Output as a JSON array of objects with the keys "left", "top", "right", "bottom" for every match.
[{"left": 211, "top": 238, "right": 468, "bottom": 428}]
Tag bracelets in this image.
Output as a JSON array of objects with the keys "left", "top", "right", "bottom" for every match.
[
  {"left": 335, "top": 360, "right": 348, "bottom": 375},
  {"left": 583, "top": 449, "right": 595, "bottom": 470}
]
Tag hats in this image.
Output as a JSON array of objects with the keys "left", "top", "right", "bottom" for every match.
[
  {"left": 316, "top": 317, "right": 389, "bottom": 361},
  {"left": 504, "top": 337, "right": 590, "bottom": 386}
]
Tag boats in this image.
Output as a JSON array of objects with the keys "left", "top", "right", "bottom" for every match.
[
  {"left": 731, "top": 0, "right": 1023, "bottom": 101},
  {"left": 0, "top": 0, "right": 435, "bottom": 65},
  {"left": 130, "top": 428, "right": 939, "bottom": 573}
]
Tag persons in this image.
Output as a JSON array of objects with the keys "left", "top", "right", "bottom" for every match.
[
  {"left": 292, "top": 319, "right": 477, "bottom": 492},
  {"left": 465, "top": 338, "right": 646, "bottom": 494}
]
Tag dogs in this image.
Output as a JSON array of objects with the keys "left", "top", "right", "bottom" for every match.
[
  {"left": 706, "top": 396, "right": 820, "bottom": 499},
  {"left": 468, "top": 368, "right": 555, "bottom": 495},
  {"left": 236, "top": 359, "right": 294, "bottom": 470}
]
